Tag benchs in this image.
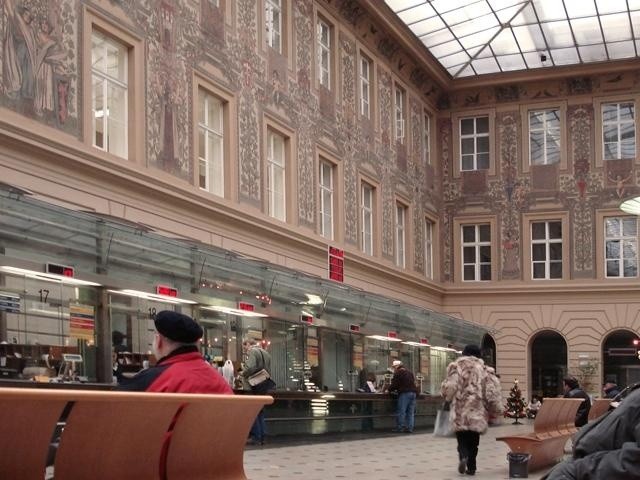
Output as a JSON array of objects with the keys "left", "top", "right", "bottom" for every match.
[
  {"left": 568, "top": 396, "right": 612, "bottom": 448},
  {"left": 0, "top": 379, "right": 275, "bottom": 480},
  {"left": 495, "top": 396, "right": 586, "bottom": 476}
]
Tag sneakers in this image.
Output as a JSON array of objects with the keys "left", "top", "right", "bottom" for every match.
[
  {"left": 459, "top": 457, "right": 468, "bottom": 474},
  {"left": 467, "top": 461, "right": 476, "bottom": 476}
]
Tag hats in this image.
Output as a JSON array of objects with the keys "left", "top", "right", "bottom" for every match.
[
  {"left": 392, "top": 360, "right": 403, "bottom": 367},
  {"left": 462, "top": 344, "right": 481, "bottom": 359},
  {"left": 155, "top": 310, "right": 204, "bottom": 344}
]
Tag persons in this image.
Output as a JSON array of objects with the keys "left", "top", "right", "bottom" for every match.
[
  {"left": 361, "top": 358, "right": 381, "bottom": 435},
  {"left": 111, "top": 329, "right": 128, "bottom": 354},
  {"left": 241, "top": 336, "right": 273, "bottom": 445},
  {"left": 435, "top": 345, "right": 502, "bottom": 475},
  {"left": 111, "top": 350, "right": 121, "bottom": 377},
  {"left": 126, "top": 312, "right": 242, "bottom": 480},
  {"left": 527, "top": 378, "right": 623, "bottom": 433},
  {"left": 388, "top": 359, "right": 417, "bottom": 433},
  {"left": 540, "top": 361, "right": 639, "bottom": 480}
]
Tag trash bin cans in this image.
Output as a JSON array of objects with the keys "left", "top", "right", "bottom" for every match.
[{"left": 507, "top": 452, "right": 529, "bottom": 478}]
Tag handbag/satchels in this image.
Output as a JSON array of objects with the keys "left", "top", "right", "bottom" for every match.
[
  {"left": 431, "top": 400, "right": 458, "bottom": 439},
  {"left": 248, "top": 368, "right": 276, "bottom": 396}
]
